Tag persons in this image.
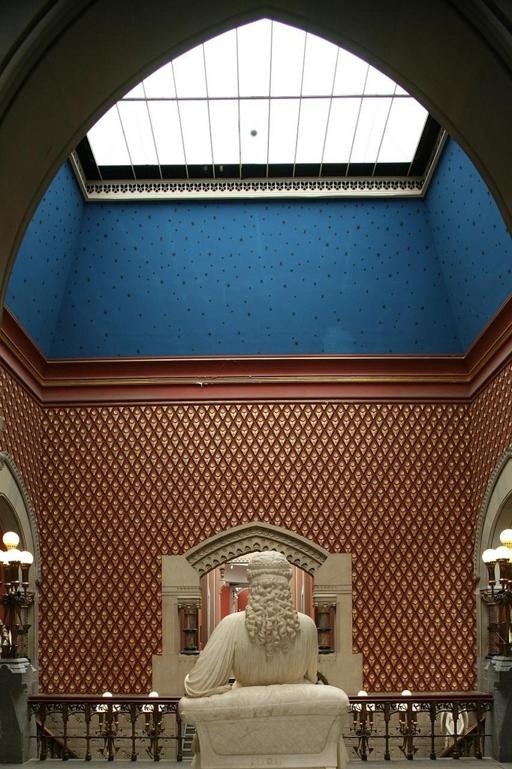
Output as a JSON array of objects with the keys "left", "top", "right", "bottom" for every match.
[{"left": 184, "top": 547, "right": 324, "bottom": 699}]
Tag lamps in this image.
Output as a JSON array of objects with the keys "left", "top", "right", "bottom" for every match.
[
  {"left": 480, "top": 529, "right": 511, "bottom": 654},
  {"left": 95, "top": 691, "right": 167, "bottom": 761},
  {"left": 1, "top": 532, "right": 34, "bottom": 657},
  {"left": 351, "top": 690, "right": 420, "bottom": 760}
]
